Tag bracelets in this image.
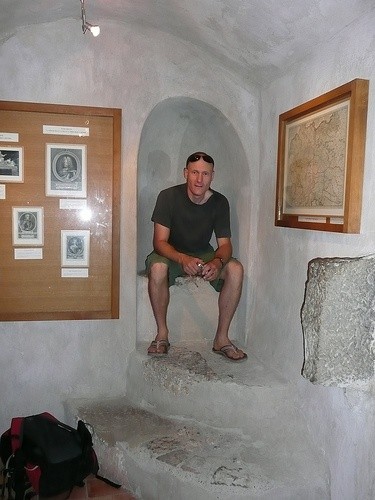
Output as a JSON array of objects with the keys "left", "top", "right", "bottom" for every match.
[{"left": 213, "top": 256, "right": 224, "bottom": 268}]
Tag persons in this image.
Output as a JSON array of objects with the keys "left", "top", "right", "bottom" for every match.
[{"left": 144, "top": 151, "right": 248, "bottom": 363}]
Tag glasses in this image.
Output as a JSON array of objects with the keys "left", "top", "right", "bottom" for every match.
[{"left": 189, "top": 154, "right": 213, "bottom": 163}]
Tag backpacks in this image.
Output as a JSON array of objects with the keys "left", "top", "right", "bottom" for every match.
[{"left": 0, "top": 411, "right": 99, "bottom": 500}]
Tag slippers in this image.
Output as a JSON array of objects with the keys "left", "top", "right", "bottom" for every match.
[
  {"left": 212, "top": 344, "right": 248, "bottom": 362},
  {"left": 148, "top": 340, "right": 170, "bottom": 358}
]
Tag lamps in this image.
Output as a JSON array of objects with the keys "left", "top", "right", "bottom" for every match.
[{"left": 80, "top": 0, "right": 101, "bottom": 36}]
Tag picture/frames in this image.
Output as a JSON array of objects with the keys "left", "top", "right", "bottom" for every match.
[
  {"left": 11, "top": 205, "right": 44, "bottom": 247},
  {"left": 60, "top": 230, "right": 91, "bottom": 268},
  {"left": 0, "top": 146, "right": 25, "bottom": 183},
  {"left": 274, "top": 79, "right": 368, "bottom": 233},
  {"left": 44, "top": 142, "right": 88, "bottom": 198}
]
